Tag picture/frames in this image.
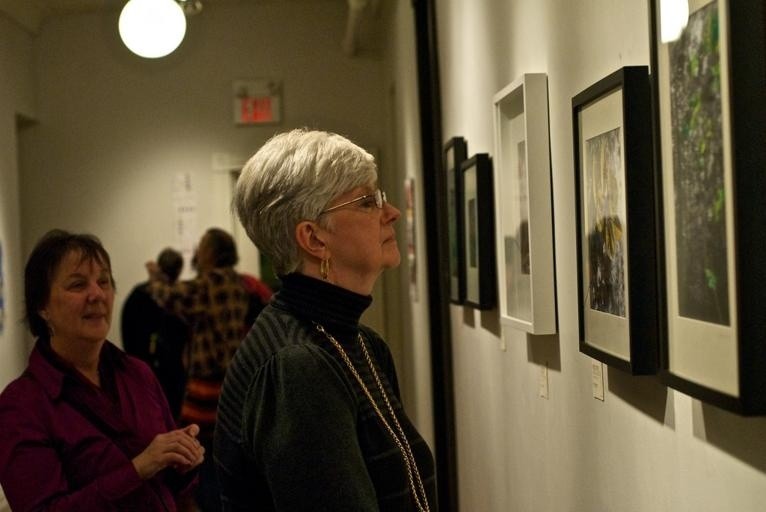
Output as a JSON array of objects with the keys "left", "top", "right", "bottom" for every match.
[
  {"left": 491, "top": 71, "right": 558, "bottom": 336},
  {"left": 439, "top": 136, "right": 496, "bottom": 313},
  {"left": 648, "top": 0, "right": 766, "bottom": 420},
  {"left": 571, "top": 66, "right": 661, "bottom": 377}
]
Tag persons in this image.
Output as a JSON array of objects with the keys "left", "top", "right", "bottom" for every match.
[
  {"left": 119, "top": 227, "right": 275, "bottom": 452},
  {"left": 215, "top": 126, "right": 435, "bottom": 512},
  {"left": 0, "top": 228, "right": 206, "bottom": 511}
]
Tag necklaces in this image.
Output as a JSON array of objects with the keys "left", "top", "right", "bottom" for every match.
[{"left": 318, "top": 322, "right": 432, "bottom": 512}]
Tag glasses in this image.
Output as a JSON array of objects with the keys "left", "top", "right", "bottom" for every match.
[{"left": 319, "top": 188, "right": 388, "bottom": 213}]
